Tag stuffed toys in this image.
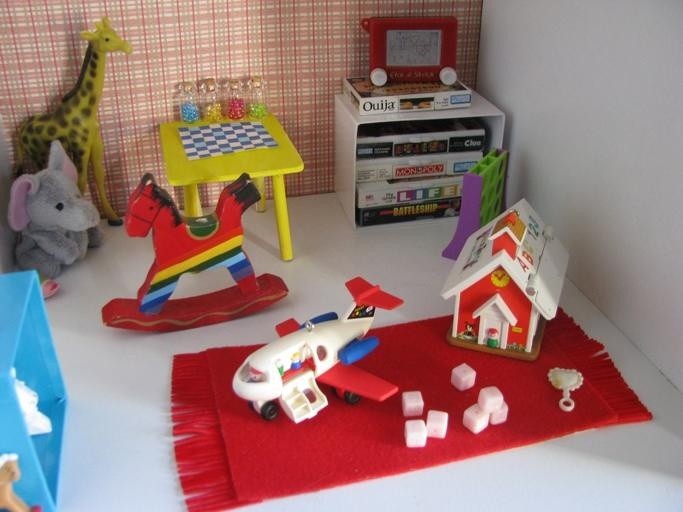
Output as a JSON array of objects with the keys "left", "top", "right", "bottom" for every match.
[
  {"left": 16, "top": 16, "right": 126, "bottom": 226},
  {"left": 5, "top": 139, "right": 105, "bottom": 302}
]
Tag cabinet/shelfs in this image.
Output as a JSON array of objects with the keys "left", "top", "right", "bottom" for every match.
[
  {"left": 334, "top": 85, "right": 506, "bottom": 230},
  {"left": 0, "top": 269, "right": 66, "bottom": 512}
]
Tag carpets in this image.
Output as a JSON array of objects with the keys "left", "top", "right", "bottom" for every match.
[{"left": 170, "top": 304, "right": 651, "bottom": 512}]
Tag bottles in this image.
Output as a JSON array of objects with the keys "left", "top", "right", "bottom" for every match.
[
  {"left": 178, "top": 77, "right": 199, "bottom": 122},
  {"left": 247, "top": 77, "right": 269, "bottom": 120},
  {"left": 199, "top": 79, "right": 223, "bottom": 122},
  {"left": 222, "top": 79, "right": 246, "bottom": 120}
]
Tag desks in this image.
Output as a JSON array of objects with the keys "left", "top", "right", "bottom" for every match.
[{"left": 159, "top": 113, "right": 304, "bottom": 261}]
